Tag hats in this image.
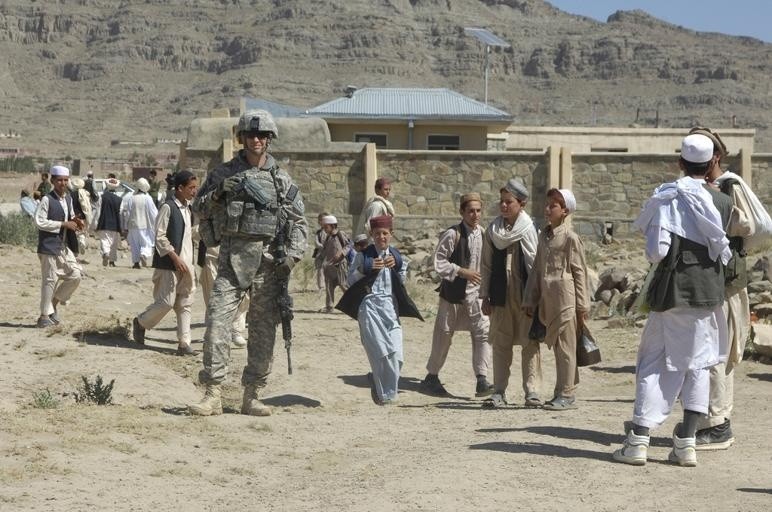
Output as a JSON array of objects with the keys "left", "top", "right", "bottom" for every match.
[
  {"left": 86, "top": 171, "right": 93, "bottom": 176},
  {"left": 135, "top": 176, "right": 151, "bottom": 193},
  {"left": 368, "top": 215, "right": 394, "bottom": 230},
  {"left": 320, "top": 215, "right": 338, "bottom": 226},
  {"left": 503, "top": 179, "right": 530, "bottom": 205},
  {"left": 680, "top": 133, "right": 716, "bottom": 166},
  {"left": 174, "top": 169, "right": 197, "bottom": 187},
  {"left": 69, "top": 177, "right": 86, "bottom": 190},
  {"left": 687, "top": 126, "right": 729, "bottom": 159},
  {"left": 557, "top": 188, "right": 577, "bottom": 216},
  {"left": 50, "top": 166, "right": 70, "bottom": 177},
  {"left": 459, "top": 191, "right": 483, "bottom": 209},
  {"left": 375, "top": 177, "right": 391, "bottom": 187}
]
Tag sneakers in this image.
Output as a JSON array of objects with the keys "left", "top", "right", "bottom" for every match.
[
  {"left": 724, "top": 418, "right": 735, "bottom": 444},
  {"left": 232, "top": 331, "right": 247, "bottom": 347},
  {"left": 668, "top": 435, "right": 698, "bottom": 467},
  {"left": 693, "top": 422, "right": 731, "bottom": 450},
  {"left": 611, "top": 429, "right": 651, "bottom": 466}
]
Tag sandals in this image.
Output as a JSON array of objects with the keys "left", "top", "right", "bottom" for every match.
[
  {"left": 541, "top": 396, "right": 578, "bottom": 410},
  {"left": 523, "top": 392, "right": 542, "bottom": 407},
  {"left": 475, "top": 379, "right": 495, "bottom": 397},
  {"left": 420, "top": 373, "right": 448, "bottom": 395},
  {"left": 481, "top": 392, "right": 508, "bottom": 406}
]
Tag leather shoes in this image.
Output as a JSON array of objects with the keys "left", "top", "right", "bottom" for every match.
[
  {"left": 49, "top": 305, "right": 61, "bottom": 324},
  {"left": 108, "top": 260, "right": 117, "bottom": 268},
  {"left": 367, "top": 371, "right": 381, "bottom": 406},
  {"left": 133, "top": 317, "right": 146, "bottom": 348},
  {"left": 103, "top": 253, "right": 109, "bottom": 267},
  {"left": 139, "top": 254, "right": 147, "bottom": 268},
  {"left": 132, "top": 261, "right": 140, "bottom": 269},
  {"left": 176, "top": 345, "right": 200, "bottom": 356},
  {"left": 36, "top": 317, "right": 54, "bottom": 328},
  {"left": 318, "top": 306, "right": 334, "bottom": 314}
]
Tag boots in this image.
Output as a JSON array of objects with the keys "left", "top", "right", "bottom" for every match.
[
  {"left": 240, "top": 382, "right": 273, "bottom": 416},
  {"left": 187, "top": 381, "right": 224, "bottom": 417}
]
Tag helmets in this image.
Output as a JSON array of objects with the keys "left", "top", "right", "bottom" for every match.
[{"left": 232, "top": 109, "right": 279, "bottom": 157}]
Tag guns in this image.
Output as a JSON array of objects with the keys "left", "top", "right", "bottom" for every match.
[{"left": 274, "top": 232, "right": 293, "bottom": 374}]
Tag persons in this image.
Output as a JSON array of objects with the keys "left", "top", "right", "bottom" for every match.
[
  {"left": 20, "top": 190, "right": 35, "bottom": 217},
  {"left": 364, "top": 179, "right": 394, "bottom": 244},
  {"left": 119, "top": 181, "right": 157, "bottom": 267},
  {"left": 35, "top": 166, "right": 83, "bottom": 327},
  {"left": 314, "top": 213, "right": 327, "bottom": 288},
  {"left": 83, "top": 171, "right": 98, "bottom": 207},
  {"left": 613, "top": 134, "right": 734, "bottom": 468},
  {"left": 146, "top": 171, "right": 161, "bottom": 203},
  {"left": 95, "top": 179, "right": 122, "bottom": 267},
  {"left": 422, "top": 193, "right": 496, "bottom": 395},
  {"left": 185, "top": 110, "right": 304, "bottom": 417},
  {"left": 479, "top": 179, "right": 538, "bottom": 407},
  {"left": 68, "top": 176, "right": 92, "bottom": 233},
  {"left": 336, "top": 216, "right": 425, "bottom": 405},
  {"left": 318, "top": 217, "right": 354, "bottom": 314},
  {"left": 523, "top": 188, "right": 596, "bottom": 410},
  {"left": 681, "top": 127, "right": 772, "bottom": 449},
  {"left": 133, "top": 169, "right": 197, "bottom": 357}
]
